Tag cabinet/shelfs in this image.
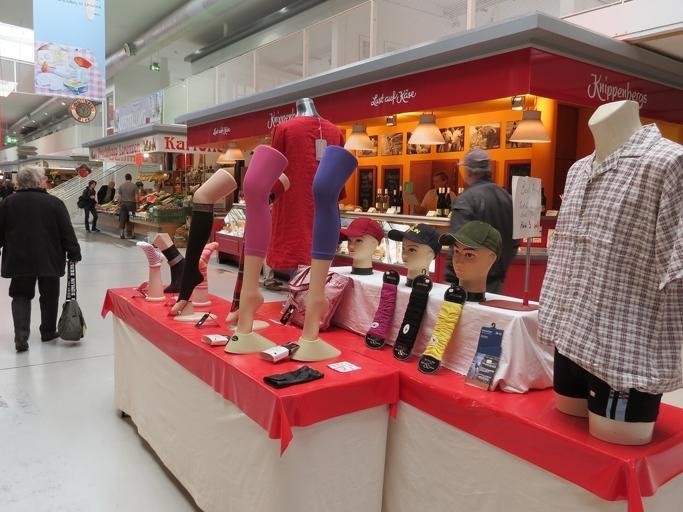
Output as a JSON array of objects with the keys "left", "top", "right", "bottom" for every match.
[{"left": 217, "top": 205, "right": 449, "bottom": 274}]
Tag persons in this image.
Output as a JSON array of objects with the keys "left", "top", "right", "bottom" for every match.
[
  {"left": 535, "top": 100, "right": 682, "bottom": 447},
  {"left": 118, "top": 174, "right": 139, "bottom": 239},
  {"left": 339, "top": 218, "right": 501, "bottom": 301},
  {"left": 0, "top": 170, "right": 16, "bottom": 200},
  {"left": 83, "top": 180, "right": 100, "bottom": 233},
  {"left": 414, "top": 172, "right": 456, "bottom": 214},
  {"left": 0, "top": 165, "right": 81, "bottom": 353},
  {"left": 444, "top": 148, "right": 520, "bottom": 294},
  {"left": 97, "top": 182, "right": 116, "bottom": 205},
  {"left": 136, "top": 97, "right": 357, "bottom": 342}
]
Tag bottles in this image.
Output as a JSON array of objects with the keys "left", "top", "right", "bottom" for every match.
[
  {"left": 375, "top": 185, "right": 404, "bottom": 214},
  {"left": 436, "top": 187, "right": 463, "bottom": 217}
]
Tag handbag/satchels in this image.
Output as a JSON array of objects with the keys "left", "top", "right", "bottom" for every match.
[{"left": 58, "top": 300, "right": 86, "bottom": 341}]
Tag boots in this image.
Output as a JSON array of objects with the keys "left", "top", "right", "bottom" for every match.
[
  {"left": 12, "top": 297, "right": 31, "bottom": 351},
  {"left": 40, "top": 300, "right": 59, "bottom": 341}
]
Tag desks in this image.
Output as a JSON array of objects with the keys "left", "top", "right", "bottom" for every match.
[
  {"left": 247, "top": 274, "right": 683, "bottom": 512},
  {"left": 101, "top": 285, "right": 399, "bottom": 512}
]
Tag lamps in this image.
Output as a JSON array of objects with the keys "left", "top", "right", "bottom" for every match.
[
  {"left": 343, "top": 121, "right": 374, "bottom": 153},
  {"left": 217, "top": 143, "right": 243, "bottom": 164},
  {"left": 407, "top": 111, "right": 446, "bottom": 147},
  {"left": 507, "top": 95, "right": 550, "bottom": 144}
]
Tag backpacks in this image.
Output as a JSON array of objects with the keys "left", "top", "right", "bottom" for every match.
[
  {"left": 77, "top": 196, "right": 84, "bottom": 208},
  {"left": 280, "top": 265, "right": 354, "bottom": 332}
]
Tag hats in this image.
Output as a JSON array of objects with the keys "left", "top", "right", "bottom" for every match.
[
  {"left": 457, "top": 149, "right": 489, "bottom": 168},
  {"left": 340, "top": 218, "right": 503, "bottom": 257}
]
